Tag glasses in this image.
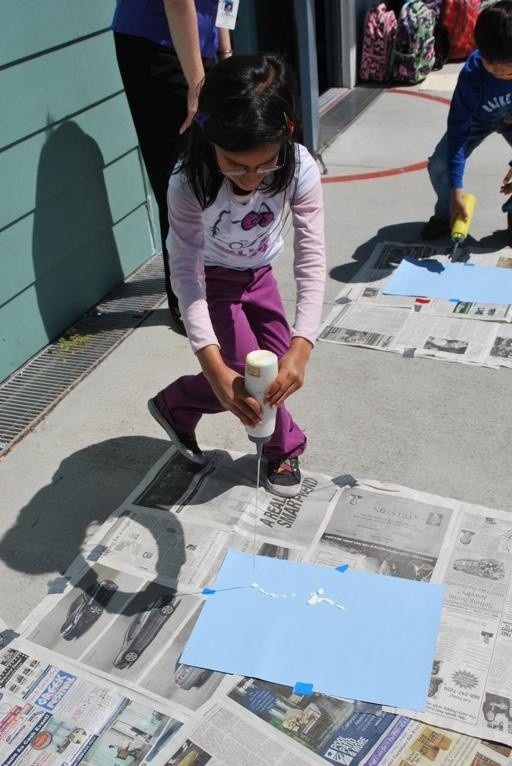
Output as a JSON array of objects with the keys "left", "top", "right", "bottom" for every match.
[{"left": 211, "top": 141, "right": 286, "bottom": 177}]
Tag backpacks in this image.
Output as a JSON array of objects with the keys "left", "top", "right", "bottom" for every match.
[{"left": 359, "top": 0, "right": 480, "bottom": 87}]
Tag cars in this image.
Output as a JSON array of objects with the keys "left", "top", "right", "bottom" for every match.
[
  {"left": 114, "top": 594, "right": 176, "bottom": 671},
  {"left": 60, "top": 579, "right": 118, "bottom": 641},
  {"left": 173, "top": 652, "right": 208, "bottom": 691}
]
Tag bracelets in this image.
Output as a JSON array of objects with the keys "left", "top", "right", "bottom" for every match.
[{"left": 217, "top": 49, "right": 233, "bottom": 55}]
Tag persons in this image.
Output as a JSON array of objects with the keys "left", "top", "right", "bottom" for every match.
[
  {"left": 224, "top": 4, "right": 232, "bottom": 11},
  {"left": 144, "top": 52, "right": 328, "bottom": 501},
  {"left": 418, "top": 0, "right": 511, "bottom": 245},
  {"left": 109, "top": 0, "right": 234, "bottom": 332}
]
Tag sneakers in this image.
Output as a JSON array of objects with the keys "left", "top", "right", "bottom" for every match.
[
  {"left": 147, "top": 394, "right": 205, "bottom": 464},
  {"left": 421, "top": 217, "right": 451, "bottom": 240},
  {"left": 263, "top": 456, "right": 303, "bottom": 498}
]
